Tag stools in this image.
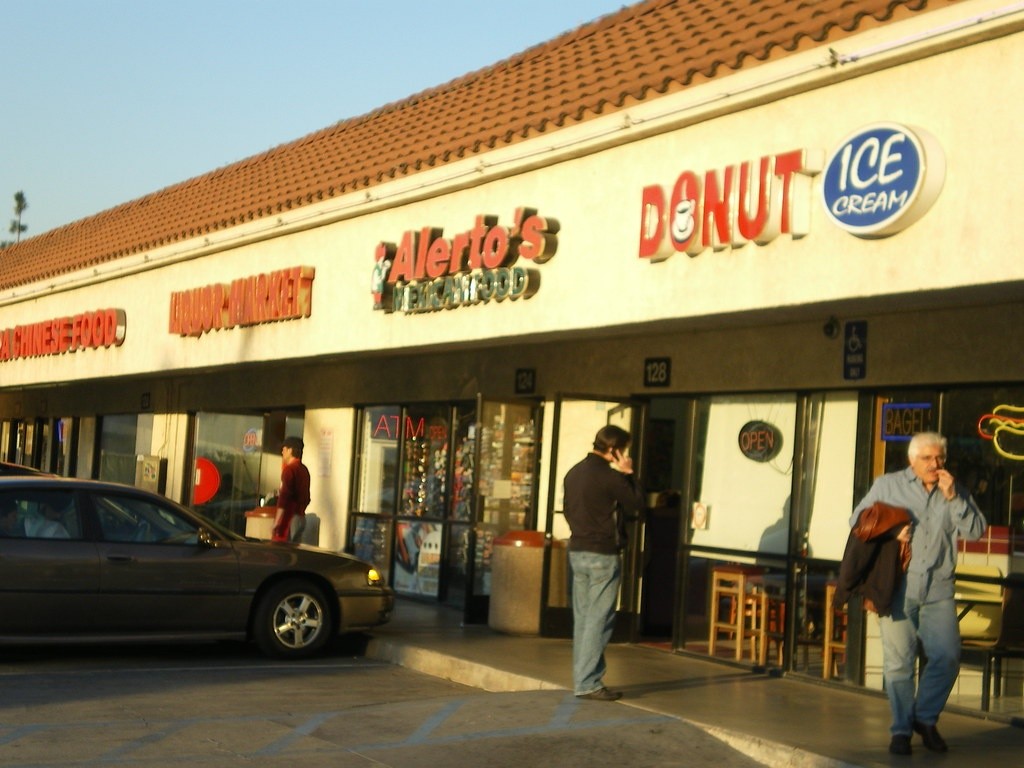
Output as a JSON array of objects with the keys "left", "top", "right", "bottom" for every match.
[
  {"left": 821, "top": 580, "right": 848, "bottom": 680},
  {"left": 706, "top": 563, "right": 784, "bottom": 665}
]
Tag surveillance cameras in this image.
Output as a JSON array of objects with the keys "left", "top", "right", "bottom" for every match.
[{"left": 823, "top": 322, "right": 838, "bottom": 338}]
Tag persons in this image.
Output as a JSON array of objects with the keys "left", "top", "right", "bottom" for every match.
[
  {"left": 271, "top": 436, "right": 310, "bottom": 542},
  {"left": 562, "top": 425, "right": 644, "bottom": 701},
  {"left": 453, "top": 452, "right": 471, "bottom": 514},
  {"left": 849, "top": 431, "right": 986, "bottom": 754}
]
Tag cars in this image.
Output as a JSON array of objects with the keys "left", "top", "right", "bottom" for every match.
[{"left": 0, "top": 460, "right": 396, "bottom": 661}]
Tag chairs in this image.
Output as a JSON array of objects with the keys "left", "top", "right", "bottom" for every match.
[
  {"left": 940, "top": 564, "right": 1024, "bottom": 711},
  {"left": 23, "top": 512, "right": 74, "bottom": 536}
]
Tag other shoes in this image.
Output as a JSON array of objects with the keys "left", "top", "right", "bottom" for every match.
[
  {"left": 912, "top": 719, "right": 948, "bottom": 753},
  {"left": 889, "top": 734, "right": 912, "bottom": 755},
  {"left": 577, "top": 688, "right": 623, "bottom": 700}
]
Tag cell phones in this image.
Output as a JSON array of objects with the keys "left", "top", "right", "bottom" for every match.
[{"left": 611, "top": 447, "right": 622, "bottom": 461}]
